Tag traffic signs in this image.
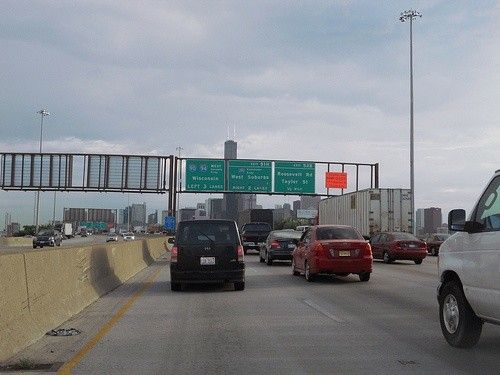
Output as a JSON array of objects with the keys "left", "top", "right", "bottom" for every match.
[
  {"left": 185, "top": 158, "right": 225, "bottom": 192},
  {"left": 226, "top": 159, "right": 272, "bottom": 193}
]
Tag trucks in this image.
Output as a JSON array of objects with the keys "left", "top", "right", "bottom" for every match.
[
  {"left": 53, "top": 223, "right": 73, "bottom": 240},
  {"left": 77, "top": 226, "right": 93, "bottom": 236},
  {"left": 310, "top": 186, "right": 415, "bottom": 240}
]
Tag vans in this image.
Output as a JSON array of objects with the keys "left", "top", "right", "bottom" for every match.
[
  {"left": 167, "top": 218, "right": 247, "bottom": 292},
  {"left": 435, "top": 168, "right": 500, "bottom": 349}
]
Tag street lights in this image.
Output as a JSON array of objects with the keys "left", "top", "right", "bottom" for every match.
[
  {"left": 34, "top": 109, "right": 51, "bottom": 234},
  {"left": 399, "top": 7, "right": 423, "bottom": 188}
]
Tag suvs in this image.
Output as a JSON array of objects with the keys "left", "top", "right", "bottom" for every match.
[{"left": 239, "top": 222, "right": 273, "bottom": 256}]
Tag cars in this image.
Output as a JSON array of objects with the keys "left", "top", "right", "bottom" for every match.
[
  {"left": 258, "top": 229, "right": 305, "bottom": 266},
  {"left": 426, "top": 233, "right": 453, "bottom": 257},
  {"left": 290, "top": 224, "right": 373, "bottom": 282},
  {"left": 31, "top": 229, "right": 62, "bottom": 249},
  {"left": 121, "top": 230, "right": 129, "bottom": 237},
  {"left": 123, "top": 232, "right": 136, "bottom": 241},
  {"left": 367, "top": 230, "right": 429, "bottom": 265},
  {"left": 81, "top": 232, "right": 88, "bottom": 237},
  {"left": 105, "top": 232, "right": 118, "bottom": 243}
]
